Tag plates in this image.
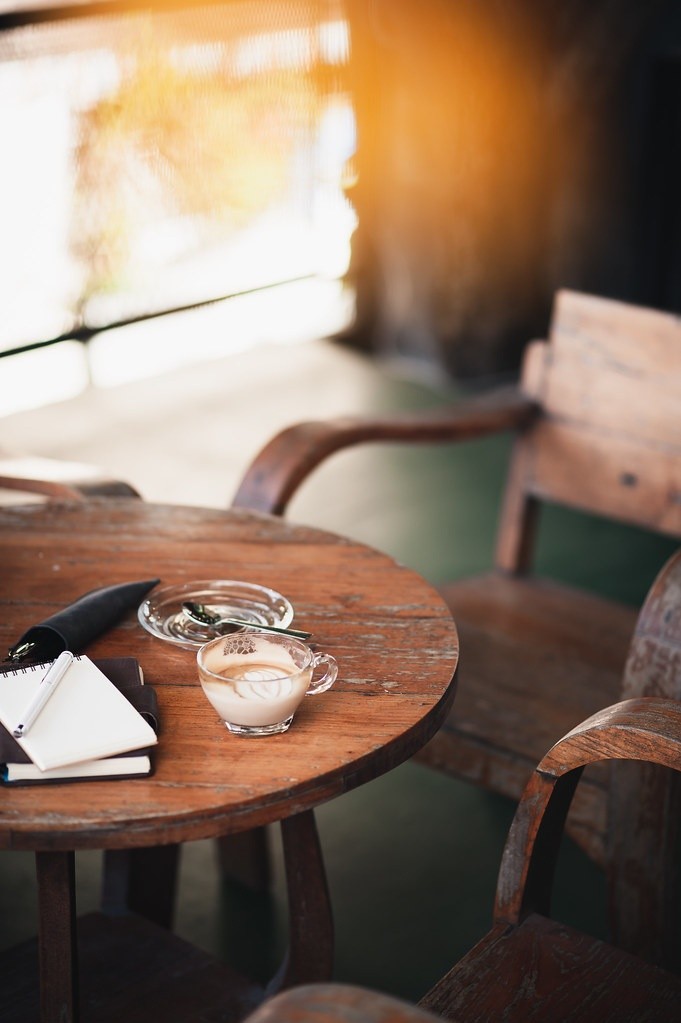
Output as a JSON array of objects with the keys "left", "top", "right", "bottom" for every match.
[{"left": 137, "top": 580, "right": 295, "bottom": 650}]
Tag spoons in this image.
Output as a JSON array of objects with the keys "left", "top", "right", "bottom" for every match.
[{"left": 181, "top": 601, "right": 313, "bottom": 641}]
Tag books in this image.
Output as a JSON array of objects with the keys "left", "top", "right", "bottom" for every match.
[{"left": 0, "top": 650, "right": 158, "bottom": 786}]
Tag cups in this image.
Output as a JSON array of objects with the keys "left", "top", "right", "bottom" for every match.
[{"left": 196, "top": 632, "right": 339, "bottom": 737}]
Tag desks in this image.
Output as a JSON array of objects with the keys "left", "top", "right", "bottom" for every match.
[{"left": 0, "top": 496, "right": 458, "bottom": 1023}]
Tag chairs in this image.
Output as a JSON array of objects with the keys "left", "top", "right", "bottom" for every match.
[
  {"left": 211, "top": 285, "right": 681, "bottom": 984},
  {"left": 246, "top": 700, "right": 681, "bottom": 1023}
]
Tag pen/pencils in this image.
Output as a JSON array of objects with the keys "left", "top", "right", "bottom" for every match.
[{"left": 12, "top": 647, "right": 74, "bottom": 742}]
